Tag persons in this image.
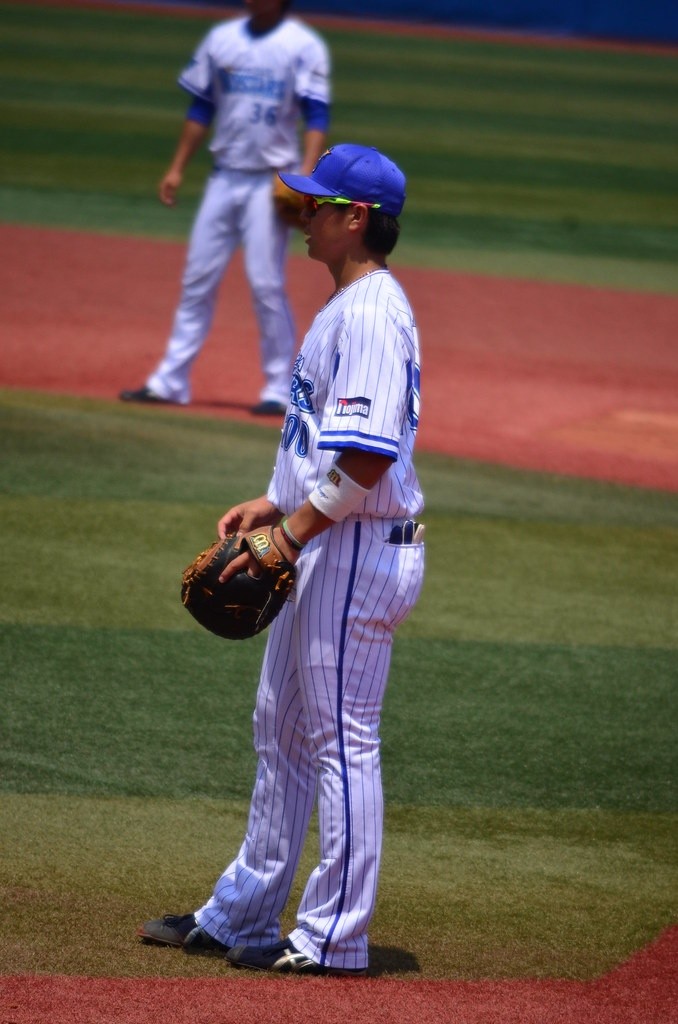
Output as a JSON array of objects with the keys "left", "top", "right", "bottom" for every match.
[
  {"left": 120, "top": 0, "right": 333, "bottom": 414},
  {"left": 140, "top": 142, "right": 427, "bottom": 971}
]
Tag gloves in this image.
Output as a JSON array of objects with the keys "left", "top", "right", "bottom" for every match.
[{"left": 389, "top": 520, "right": 425, "bottom": 545}]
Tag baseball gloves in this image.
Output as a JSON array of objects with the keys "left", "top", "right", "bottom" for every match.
[{"left": 180, "top": 524, "right": 298, "bottom": 641}]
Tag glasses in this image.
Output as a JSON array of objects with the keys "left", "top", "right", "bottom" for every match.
[{"left": 303, "top": 194, "right": 381, "bottom": 217}]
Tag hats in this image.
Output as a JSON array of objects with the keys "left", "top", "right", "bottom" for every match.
[{"left": 278, "top": 144, "right": 406, "bottom": 219}]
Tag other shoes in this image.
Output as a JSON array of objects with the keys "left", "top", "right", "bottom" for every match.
[
  {"left": 251, "top": 402, "right": 287, "bottom": 414},
  {"left": 121, "top": 387, "right": 170, "bottom": 403}
]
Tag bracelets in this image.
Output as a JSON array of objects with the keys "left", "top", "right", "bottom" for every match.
[{"left": 280, "top": 520, "right": 308, "bottom": 551}]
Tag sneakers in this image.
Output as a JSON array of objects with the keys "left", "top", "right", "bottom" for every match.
[
  {"left": 138, "top": 914, "right": 229, "bottom": 953},
  {"left": 224, "top": 937, "right": 367, "bottom": 975}
]
